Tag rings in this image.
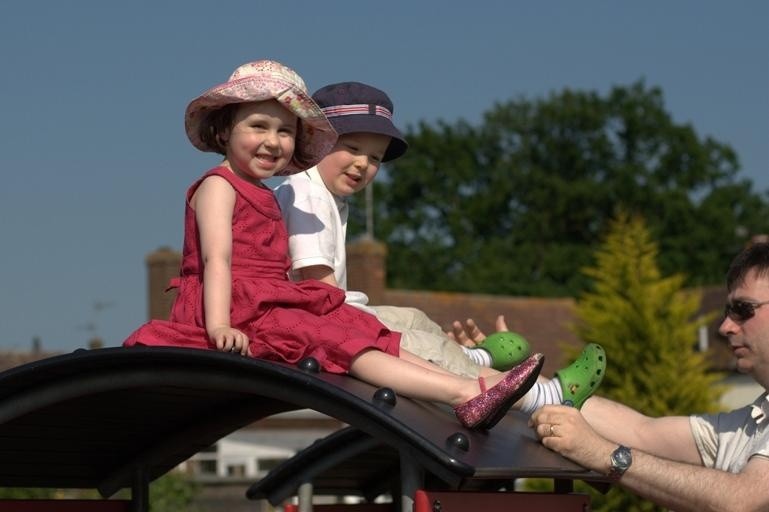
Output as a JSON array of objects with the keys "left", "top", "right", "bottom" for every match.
[{"left": 549, "top": 425, "right": 555, "bottom": 436}]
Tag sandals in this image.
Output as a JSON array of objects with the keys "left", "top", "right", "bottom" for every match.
[
  {"left": 474, "top": 331, "right": 533, "bottom": 372},
  {"left": 556, "top": 341, "right": 608, "bottom": 413}
]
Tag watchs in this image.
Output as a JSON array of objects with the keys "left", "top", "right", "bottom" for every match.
[{"left": 602, "top": 443, "right": 632, "bottom": 484}]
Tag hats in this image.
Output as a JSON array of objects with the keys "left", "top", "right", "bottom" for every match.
[
  {"left": 311, "top": 82, "right": 407, "bottom": 163},
  {"left": 186, "top": 59, "right": 338, "bottom": 175}
]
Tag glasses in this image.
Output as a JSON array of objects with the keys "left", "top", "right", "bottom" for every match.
[{"left": 725, "top": 301, "right": 769, "bottom": 322}]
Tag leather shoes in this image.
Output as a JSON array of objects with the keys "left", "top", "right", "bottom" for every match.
[{"left": 456, "top": 354, "right": 544, "bottom": 429}]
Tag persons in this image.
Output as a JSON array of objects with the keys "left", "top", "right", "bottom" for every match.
[
  {"left": 446, "top": 241, "right": 768, "bottom": 512},
  {"left": 124, "top": 60, "right": 544, "bottom": 432},
  {"left": 271, "top": 82, "right": 607, "bottom": 418}
]
